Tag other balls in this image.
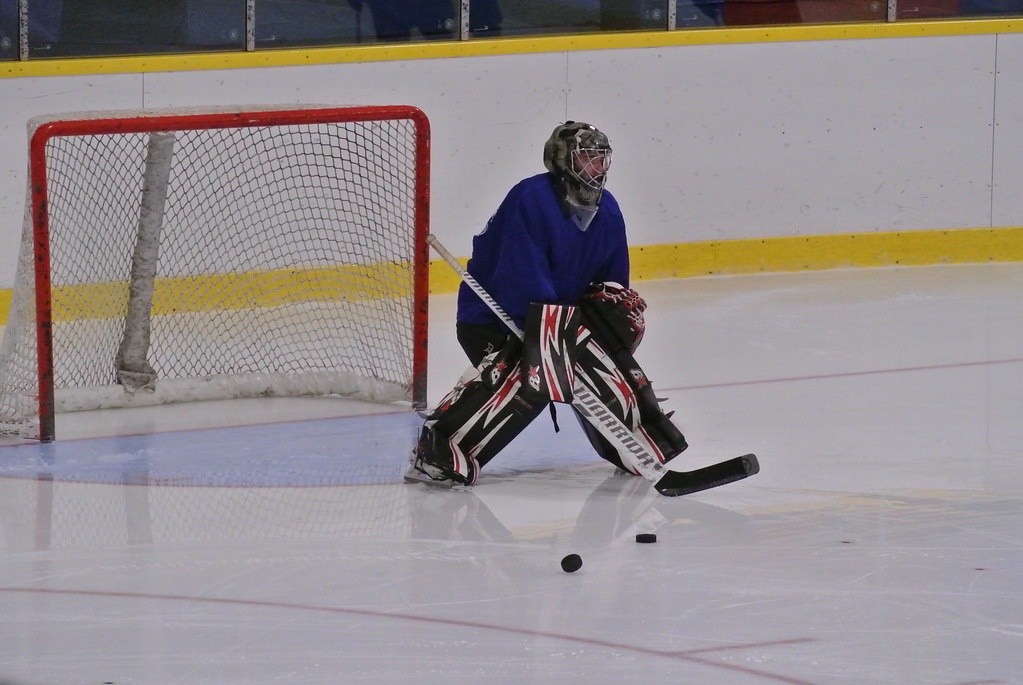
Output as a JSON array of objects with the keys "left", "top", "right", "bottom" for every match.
[
  {"left": 636, "top": 534, "right": 657, "bottom": 544},
  {"left": 561, "top": 554, "right": 583, "bottom": 573}
]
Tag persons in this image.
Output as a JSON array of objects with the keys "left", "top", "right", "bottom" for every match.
[
  {"left": 409, "top": 121, "right": 686, "bottom": 490},
  {"left": 349, "top": 0, "right": 503, "bottom": 39}
]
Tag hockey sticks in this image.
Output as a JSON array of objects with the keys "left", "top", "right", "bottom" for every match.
[{"left": 426, "top": 233, "right": 761, "bottom": 496}]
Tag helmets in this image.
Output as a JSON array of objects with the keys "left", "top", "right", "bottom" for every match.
[{"left": 544, "top": 120, "right": 612, "bottom": 206}]
